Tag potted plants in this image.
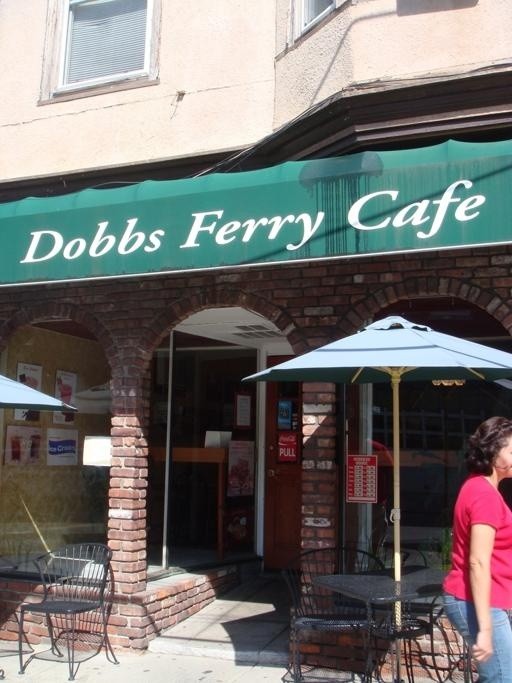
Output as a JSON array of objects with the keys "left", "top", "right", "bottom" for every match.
[
  {"left": 276, "top": 399, "right": 293, "bottom": 430},
  {"left": 233, "top": 392, "right": 255, "bottom": 430}
]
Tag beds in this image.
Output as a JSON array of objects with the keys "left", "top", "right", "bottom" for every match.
[
  {"left": 241, "top": 315, "right": 512, "bottom": 680},
  {"left": 0, "top": 374, "right": 78, "bottom": 412}
]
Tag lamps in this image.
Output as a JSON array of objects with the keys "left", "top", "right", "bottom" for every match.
[
  {"left": 281, "top": 547, "right": 394, "bottom": 683},
  {"left": 19, "top": 543, "right": 119, "bottom": 680}
]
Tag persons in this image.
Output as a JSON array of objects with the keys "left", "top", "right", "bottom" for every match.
[
  {"left": 371, "top": 441, "right": 395, "bottom": 561},
  {"left": 441, "top": 416, "right": 512, "bottom": 683}
]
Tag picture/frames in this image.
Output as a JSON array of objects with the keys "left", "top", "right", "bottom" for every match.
[{"left": 312, "top": 568, "right": 444, "bottom": 683}]
